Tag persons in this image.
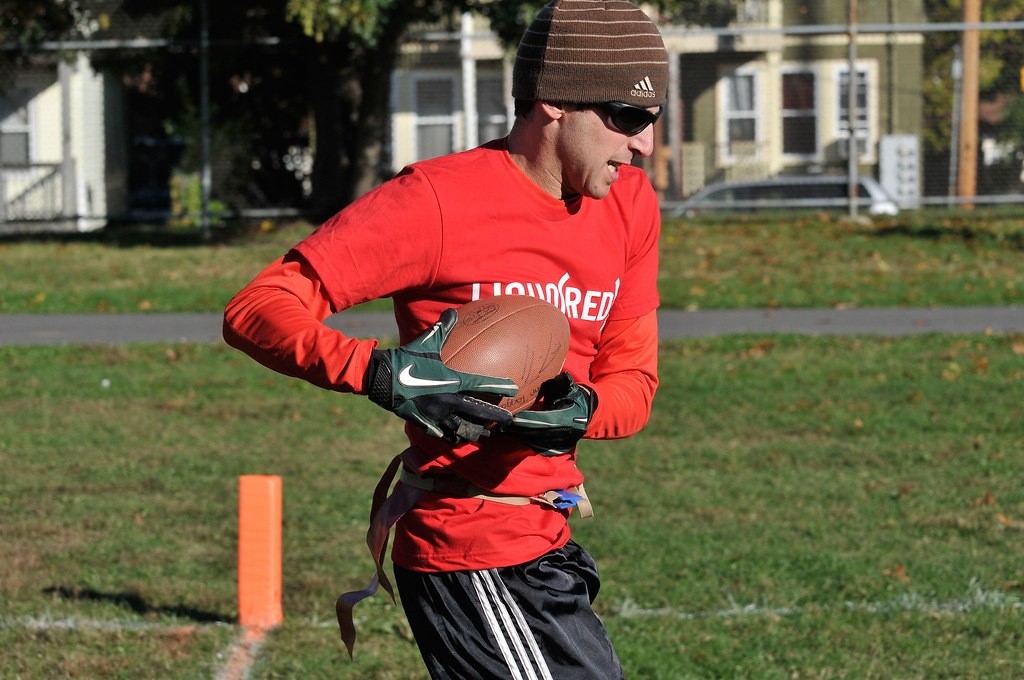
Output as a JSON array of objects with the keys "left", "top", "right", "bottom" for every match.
[{"left": 222, "top": 0, "right": 671, "bottom": 680}]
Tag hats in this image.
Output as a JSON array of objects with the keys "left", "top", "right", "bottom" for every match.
[{"left": 512, "top": 0, "right": 668, "bottom": 107}]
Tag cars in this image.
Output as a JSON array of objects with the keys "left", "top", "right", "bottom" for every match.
[{"left": 660, "top": 174, "right": 901, "bottom": 220}]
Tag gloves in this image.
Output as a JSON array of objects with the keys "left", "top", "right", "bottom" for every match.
[
  {"left": 366, "top": 307, "right": 519, "bottom": 445},
  {"left": 499, "top": 371, "right": 594, "bottom": 457}
]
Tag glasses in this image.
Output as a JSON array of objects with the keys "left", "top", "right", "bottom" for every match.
[{"left": 592, "top": 101, "right": 664, "bottom": 136}]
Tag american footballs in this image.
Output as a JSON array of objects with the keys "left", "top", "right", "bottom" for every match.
[{"left": 440, "top": 296, "right": 571, "bottom": 416}]
